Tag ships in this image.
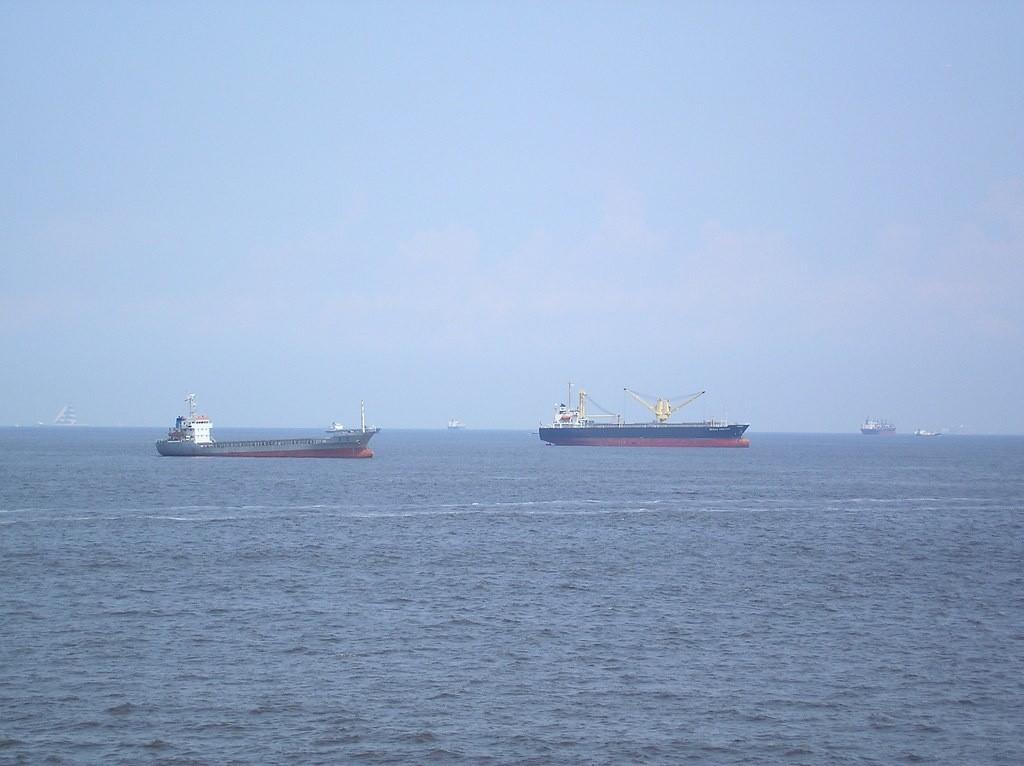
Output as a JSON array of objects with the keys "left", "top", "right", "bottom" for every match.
[
  {"left": 539, "top": 385, "right": 750, "bottom": 451},
  {"left": 157, "top": 390, "right": 381, "bottom": 462},
  {"left": 861, "top": 418, "right": 897, "bottom": 434},
  {"left": 38, "top": 402, "right": 92, "bottom": 427}
]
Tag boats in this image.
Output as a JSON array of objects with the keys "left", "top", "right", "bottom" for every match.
[
  {"left": 913, "top": 428, "right": 943, "bottom": 437},
  {"left": 447, "top": 419, "right": 464, "bottom": 429}
]
